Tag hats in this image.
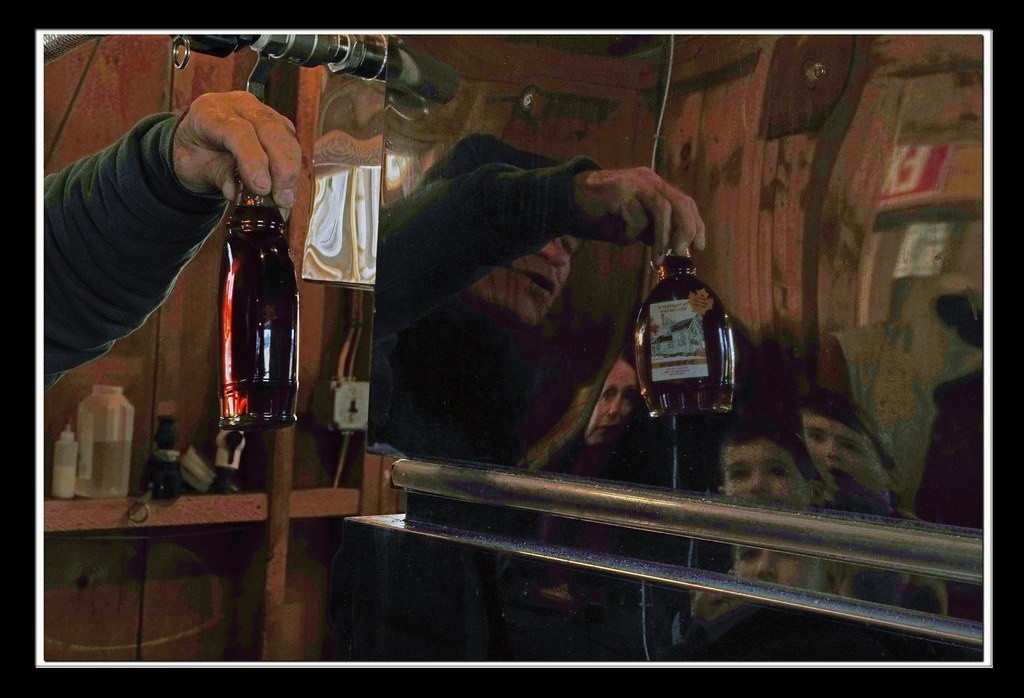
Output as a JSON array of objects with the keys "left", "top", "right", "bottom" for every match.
[{"left": 415, "top": 133, "right": 562, "bottom": 190}]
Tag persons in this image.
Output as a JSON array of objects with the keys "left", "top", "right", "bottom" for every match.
[
  {"left": 369, "top": 132, "right": 951, "bottom": 657},
  {"left": 43, "top": 89, "right": 302, "bottom": 391}
]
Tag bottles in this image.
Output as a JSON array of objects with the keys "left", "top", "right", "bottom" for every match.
[
  {"left": 220, "top": 168, "right": 299, "bottom": 430},
  {"left": 635, "top": 248, "right": 736, "bottom": 418},
  {"left": 79, "top": 383, "right": 134, "bottom": 499},
  {"left": 52, "top": 421, "right": 77, "bottom": 498}
]
